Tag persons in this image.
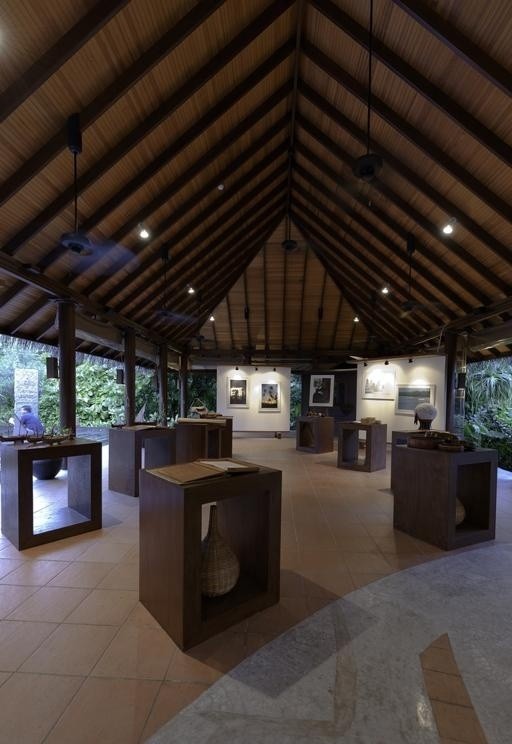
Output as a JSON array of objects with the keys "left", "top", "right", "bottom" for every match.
[{"left": 0, "top": 405, "right": 44, "bottom": 442}]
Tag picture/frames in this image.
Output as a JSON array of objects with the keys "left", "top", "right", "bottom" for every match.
[
  {"left": 394, "top": 384, "right": 436, "bottom": 415},
  {"left": 361, "top": 368, "right": 396, "bottom": 400},
  {"left": 258, "top": 381, "right": 281, "bottom": 412},
  {"left": 227, "top": 377, "right": 249, "bottom": 409},
  {"left": 309, "top": 375, "right": 335, "bottom": 407}
]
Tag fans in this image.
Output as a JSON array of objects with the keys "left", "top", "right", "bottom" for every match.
[
  {"left": 22, "top": 125, "right": 139, "bottom": 275},
  {"left": 139, "top": 246, "right": 198, "bottom": 324},
  {"left": 382, "top": 238, "right": 441, "bottom": 318}
]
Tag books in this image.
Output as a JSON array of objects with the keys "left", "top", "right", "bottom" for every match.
[
  {"left": 177, "top": 417, "right": 230, "bottom": 426},
  {"left": 122, "top": 425, "right": 158, "bottom": 431},
  {"left": 146, "top": 457, "right": 260, "bottom": 485}
]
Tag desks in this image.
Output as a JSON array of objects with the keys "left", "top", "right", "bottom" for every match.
[
  {"left": 139, "top": 458, "right": 282, "bottom": 651},
  {"left": 1, "top": 437, "right": 102, "bottom": 551},
  {"left": 296, "top": 416, "right": 334, "bottom": 453},
  {"left": 392, "top": 429, "right": 498, "bottom": 550},
  {"left": 338, "top": 421, "right": 387, "bottom": 472}
]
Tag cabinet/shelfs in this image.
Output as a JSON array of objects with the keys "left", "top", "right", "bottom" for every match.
[{"left": 109, "top": 414, "right": 233, "bottom": 497}]
